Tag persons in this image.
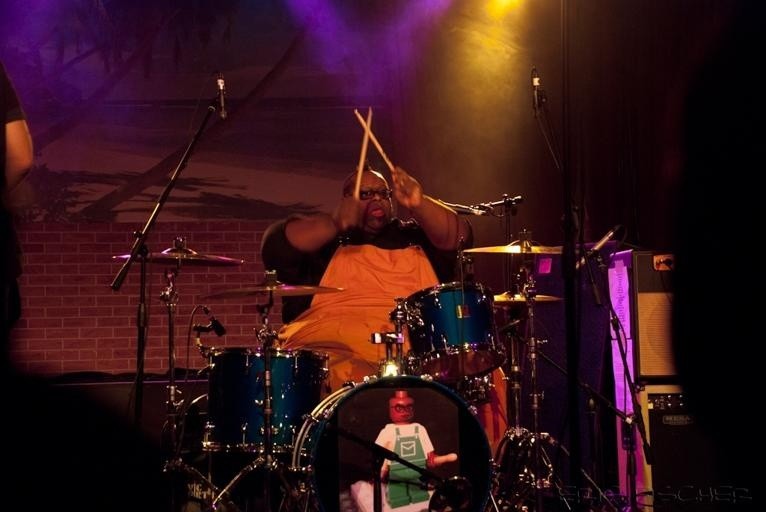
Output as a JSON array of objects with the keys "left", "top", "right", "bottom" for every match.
[
  {"left": 260, "top": 159, "right": 509, "bottom": 464},
  {"left": 0, "top": 61, "right": 34, "bottom": 337}
]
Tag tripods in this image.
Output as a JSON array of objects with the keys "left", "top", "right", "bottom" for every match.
[
  {"left": 492, "top": 258, "right": 620, "bottom": 512},
  {"left": 143, "top": 269, "right": 236, "bottom": 512},
  {"left": 212, "top": 306, "right": 313, "bottom": 512}
]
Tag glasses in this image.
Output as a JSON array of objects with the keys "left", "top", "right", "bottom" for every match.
[{"left": 360, "top": 190, "right": 392, "bottom": 200}]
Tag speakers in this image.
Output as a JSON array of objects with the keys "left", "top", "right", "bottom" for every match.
[{"left": 608, "top": 249, "right": 702, "bottom": 512}]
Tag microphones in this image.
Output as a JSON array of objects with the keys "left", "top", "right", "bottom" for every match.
[
  {"left": 436, "top": 199, "right": 486, "bottom": 217},
  {"left": 216, "top": 71, "right": 228, "bottom": 121},
  {"left": 575, "top": 223, "right": 621, "bottom": 269},
  {"left": 201, "top": 306, "right": 226, "bottom": 336},
  {"left": 530, "top": 68, "right": 542, "bottom": 118}
]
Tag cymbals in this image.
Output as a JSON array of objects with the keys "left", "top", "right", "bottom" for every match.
[
  {"left": 199, "top": 280, "right": 346, "bottom": 299},
  {"left": 493, "top": 290, "right": 561, "bottom": 303},
  {"left": 462, "top": 243, "right": 564, "bottom": 256},
  {"left": 112, "top": 248, "right": 244, "bottom": 267}
]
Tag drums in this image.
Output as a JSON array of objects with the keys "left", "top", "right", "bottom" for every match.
[
  {"left": 201, "top": 344, "right": 329, "bottom": 456},
  {"left": 403, "top": 281, "right": 506, "bottom": 388},
  {"left": 286, "top": 375, "right": 495, "bottom": 512}
]
